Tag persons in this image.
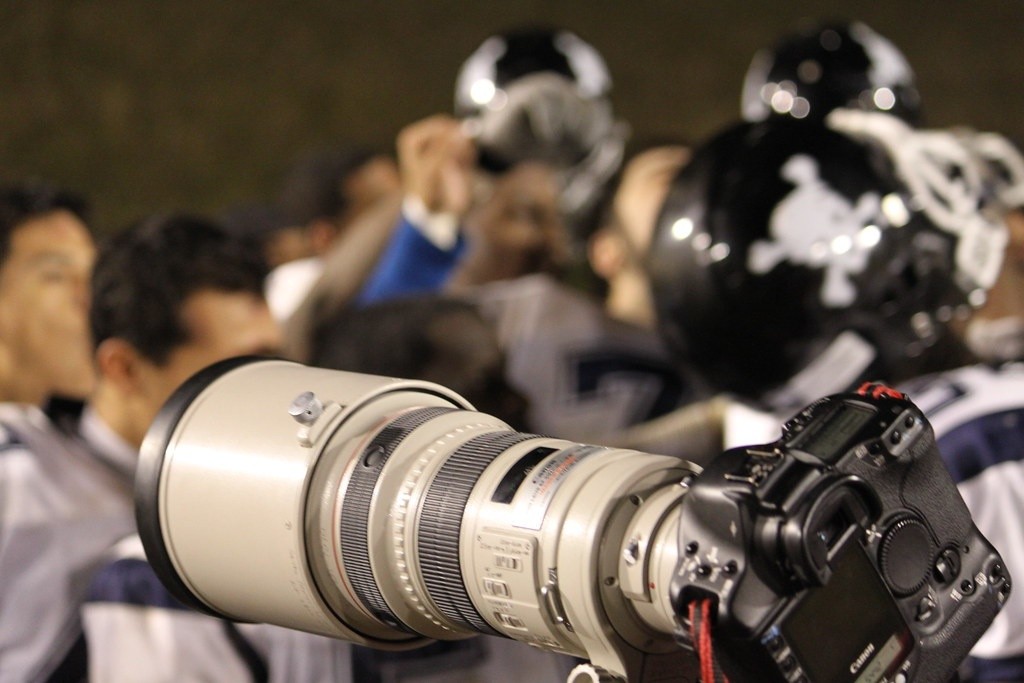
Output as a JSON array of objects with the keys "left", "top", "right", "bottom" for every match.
[{"left": 0, "top": 29, "right": 1024, "bottom": 683}]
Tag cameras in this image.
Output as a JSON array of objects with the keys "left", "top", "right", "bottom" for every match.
[{"left": 133, "top": 353, "right": 1012, "bottom": 683}]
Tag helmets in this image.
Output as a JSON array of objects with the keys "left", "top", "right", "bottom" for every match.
[
  {"left": 739, "top": 20, "right": 913, "bottom": 124},
  {"left": 643, "top": 120, "right": 981, "bottom": 412},
  {"left": 451, "top": 28, "right": 613, "bottom": 112}
]
